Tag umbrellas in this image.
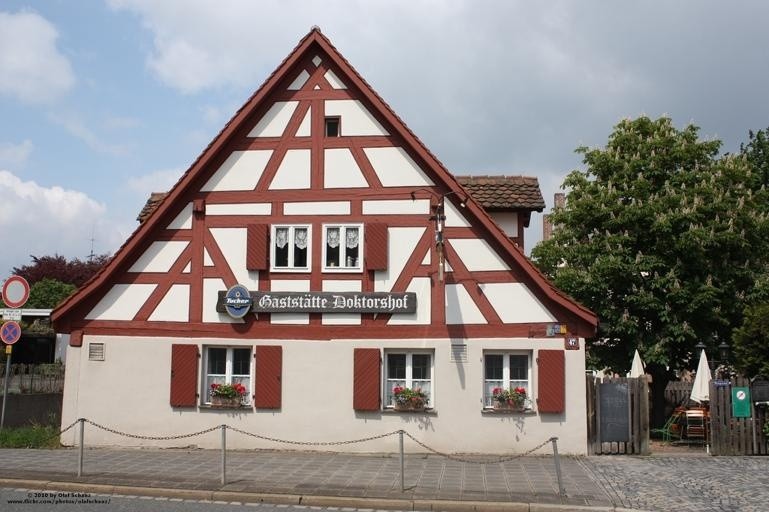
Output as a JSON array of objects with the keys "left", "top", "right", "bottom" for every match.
[
  {"left": 630, "top": 349, "right": 645, "bottom": 377},
  {"left": 690, "top": 349, "right": 713, "bottom": 405}
]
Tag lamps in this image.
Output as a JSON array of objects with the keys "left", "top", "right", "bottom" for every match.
[{"left": 410, "top": 188, "right": 469, "bottom": 284}]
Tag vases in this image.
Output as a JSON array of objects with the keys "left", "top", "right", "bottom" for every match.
[
  {"left": 396, "top": 399, "right": 426, "bottom": 413},
  {"left": 210, "top": 396, "right": 242, "bottom": 409},
  {"left": 493, "top": 398, "right": 524, "bottom": 411}
]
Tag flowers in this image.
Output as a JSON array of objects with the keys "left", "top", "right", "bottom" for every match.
[
  {"left": 209, "top": 384, "right": 250, "bottom": 399},
  {"left": 392, "top": 385, "right": 430, "bottom": 408},
  {"left": 492, "top": 386, "right": 528, "bottom": 408}
]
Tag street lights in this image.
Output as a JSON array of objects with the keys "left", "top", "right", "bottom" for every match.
[{"left": 694, "top": 338, "right": 730, "bottom": 380}]
